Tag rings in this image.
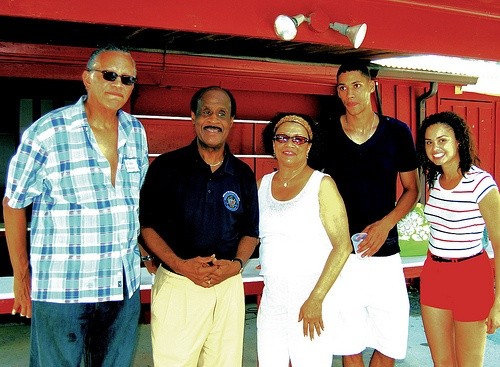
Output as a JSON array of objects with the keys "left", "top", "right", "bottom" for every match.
[{"left": 207, "top": 280, "right": 211, "bottom": 284}]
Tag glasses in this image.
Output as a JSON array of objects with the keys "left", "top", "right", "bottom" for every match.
[
  {"left": 86, "top": 68, "right": 138, "bottom": 86},
  {"left": 272, "top": 133, "right": 313, "bottom": 145}
]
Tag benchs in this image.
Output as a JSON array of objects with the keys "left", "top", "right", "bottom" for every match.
[{"left": 0, "top": 241, "right": 494, "bottom": 315}]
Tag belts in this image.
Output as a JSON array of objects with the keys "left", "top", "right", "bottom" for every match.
[
  {"left": 430, "top": 252, "right": 484, "bottom": 263},
  {"left": 160, "top": 262, "right": 186, "bottom": 276}
]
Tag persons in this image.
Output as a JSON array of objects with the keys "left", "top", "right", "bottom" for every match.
[
  {"left": 256, "top": 112, "right": 353, "bottom": 367},
  {"left": 2, "top": 46, "right": 157, "bottom": 367},
  {"left": 307, "top": 62, "right": 421, "bottom": 367},
  {"left": 416, "top": 111, "right": 500, "bottom": 367},
  {"left": 139, "top": 85, "right": 259, "bottom": 367}
]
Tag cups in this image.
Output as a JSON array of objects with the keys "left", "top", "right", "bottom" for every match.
[{"left": 351, "top": 232, "right": 369, "bottom": 259}]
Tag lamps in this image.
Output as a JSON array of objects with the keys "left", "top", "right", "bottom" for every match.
[
  {"left": 273, "top": 13, "right": 310, "bottom": 41},
  {"left": 330, "top": 21, "right": 368, "bottom": 49}
]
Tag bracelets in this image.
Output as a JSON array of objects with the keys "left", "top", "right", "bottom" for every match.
[
  {"left": 141, "top": 254, "right": 154, "bottom": 261},
  {"left": 232, "top": 257, "right": 243, "bottom": 274}
]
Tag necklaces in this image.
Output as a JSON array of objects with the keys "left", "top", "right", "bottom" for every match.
[
  {"left": 279, "top": 172, "right": 300, "bottom": 188},
  {"left": 209, "top": 160, "right": 223, "bottom": 166},
  {"left": 346, "top": 114, "right": 375, "bottom": 143}
]
